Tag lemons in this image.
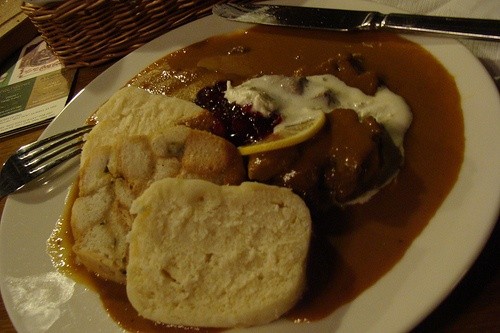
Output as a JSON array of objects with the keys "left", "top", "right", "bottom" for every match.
[{"left": 235, "top": 111, "right": 325, "bottom": 155}]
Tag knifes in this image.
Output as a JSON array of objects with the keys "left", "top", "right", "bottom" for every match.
[{"left": 212, "top": 1, "right": 500, "bottom": 41}]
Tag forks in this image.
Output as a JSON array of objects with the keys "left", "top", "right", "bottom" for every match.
[{"left": 0, "top": 125, "right": 95, "bottom": 200}]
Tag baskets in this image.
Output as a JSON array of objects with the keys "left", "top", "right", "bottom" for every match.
[{"left": 20, "top": 0, "right": 252, "bottom": 69}]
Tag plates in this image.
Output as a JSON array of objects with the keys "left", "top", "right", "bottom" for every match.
[{"left": 0, "top": 1, "right": 500, "bottom": 332}]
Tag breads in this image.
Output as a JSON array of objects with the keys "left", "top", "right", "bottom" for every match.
[{"left": 127, "top": 178, "right": 312, "bottom": 326}]
